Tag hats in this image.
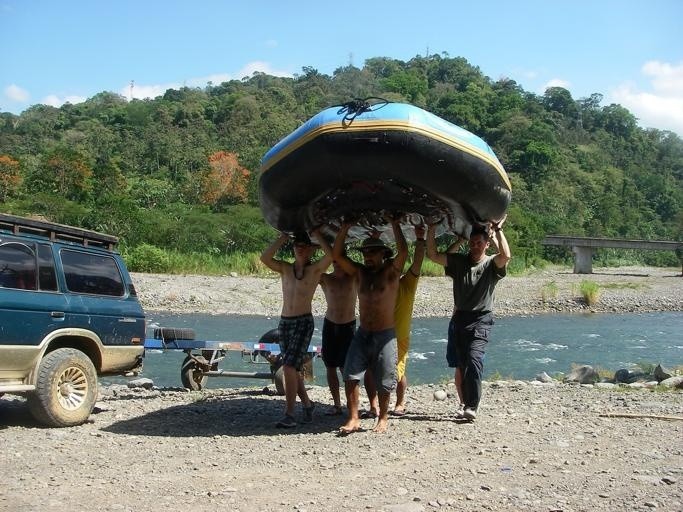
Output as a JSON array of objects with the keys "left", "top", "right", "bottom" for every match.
[
  {"left": 288, "top": 231, "right": 320, "bottom": 249},
  {"left": 351, "top": 238, "right": 394, "bottom": 258}
]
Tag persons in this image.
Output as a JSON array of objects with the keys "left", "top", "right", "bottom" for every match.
[
  {"left": 259, "top": 225, "right": 334, "bottom": 430},
  {"left": 331, "top": 214, "right": 409, "bottom": 435},
  {"left": 422, "top": 215, "right": 511, "bottom": 421},
  {"left": 317, "top": 247, "right": 362, "bottom": 415},
  {"left": 361, "top": 225, "right": 425, "bottom": 419},
  {"left": 441, "top": 226, "right": 499, "bottom": 418}
]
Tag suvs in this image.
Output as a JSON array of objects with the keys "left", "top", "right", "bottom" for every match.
[{"left": 0, "top": 210, "right": 145, "bottom": 429}]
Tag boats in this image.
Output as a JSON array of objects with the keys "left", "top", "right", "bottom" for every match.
[{"left": 256, "top": 101, "right": 511, "bottom": 250}]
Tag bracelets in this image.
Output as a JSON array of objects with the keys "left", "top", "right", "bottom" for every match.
[
  {"left": 495, "top": 228, "right": 503, "bottom": 232},
  {"left": 417, "top": 238, "right": 424, "bottom": 242}
]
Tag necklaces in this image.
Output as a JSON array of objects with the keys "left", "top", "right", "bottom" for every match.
[{"left": 292, "top": 260, "right": 305, "bottom": 280}]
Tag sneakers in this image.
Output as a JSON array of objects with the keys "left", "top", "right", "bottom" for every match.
[
  {"left": 302, "top": 400, "right": 317, "bottom": 424},
  {"left": 274, "top": 415, "right": 296, "bottom": 427},
  {"left": 456, "top": 403, "right": 466, "bottom": 416},
  {"left": 463, "top": 407, "right": 476, "bottom": 420},
  {"left": 324, "top": 405, "right": 343, "bottom": 416}
]
